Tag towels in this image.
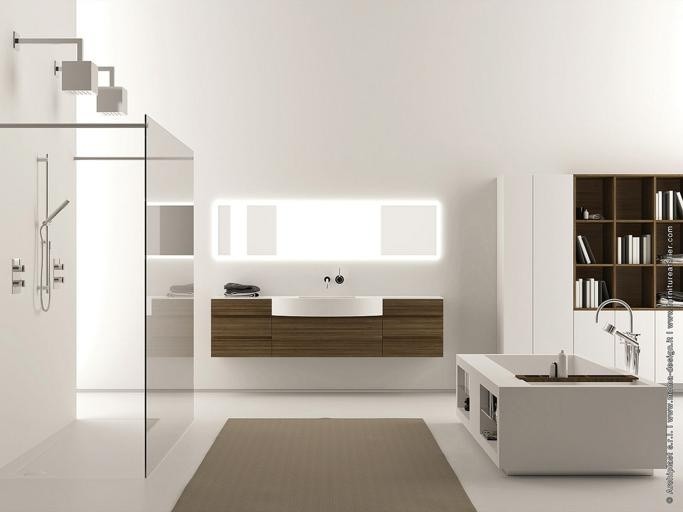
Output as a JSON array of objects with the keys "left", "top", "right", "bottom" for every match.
[
  {"left": 225, "top": 281, "right": 261, "bottom": 293},
  {"left": 170, "top": 283, "right": 193, "bottom": 294}
]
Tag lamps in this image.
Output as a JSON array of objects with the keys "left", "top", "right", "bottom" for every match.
[
  {"left": 10, "top": 29, "right": 99, "bottom": 97},
  {"left": 52, "top": 58, "right": 129, "bottom": 118}
]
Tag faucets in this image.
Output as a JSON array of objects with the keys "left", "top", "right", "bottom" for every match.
[
  {"left": 323, "top": 276, "right": 330, "bottom": 289},
  {"left": 595, "top": 298, "right": 634, "bottom": 373}
]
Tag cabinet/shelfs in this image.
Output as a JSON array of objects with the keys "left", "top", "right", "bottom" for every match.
[
  {"left": 455, "top": 366, "right": 503, "bottom": 459},
  {"left": 571, "top": 172, "right": 683, "bottom": 309}
]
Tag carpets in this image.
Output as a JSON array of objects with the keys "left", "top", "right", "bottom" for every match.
[{"left": 173, "top": 416, "right": 474, "bottom": 511}]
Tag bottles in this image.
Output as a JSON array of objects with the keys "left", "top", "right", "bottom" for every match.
[
  {"left": 583, "top": 208, "right": 589, "bottom": 220},
  {"left": 548, "top": 361, "right": 557, "bottom": 378},
  {"left": 556, "top": 348, "right": 568, "bottom": 378}
]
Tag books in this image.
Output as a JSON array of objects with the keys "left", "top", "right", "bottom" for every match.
[{"left": 575, "top": 191, "right": 682, "bottom": 308}]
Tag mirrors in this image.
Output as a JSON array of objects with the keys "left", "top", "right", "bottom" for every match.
[{"left": 209, "top": 196, "right": 445, "bottom": 260}]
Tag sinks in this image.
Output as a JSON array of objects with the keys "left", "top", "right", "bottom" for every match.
[
  {"left": 299, "top": 297, "right": 355, "bottom": 299},
  {"left": 483, "top": 354, "right": 628, "bottom": 381}
]
